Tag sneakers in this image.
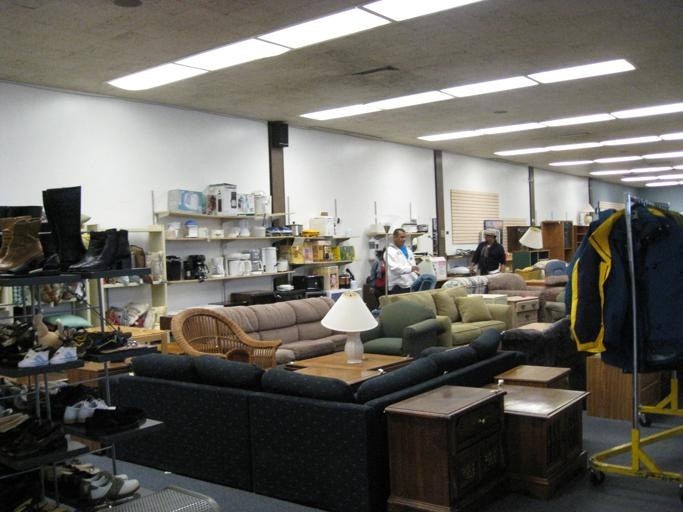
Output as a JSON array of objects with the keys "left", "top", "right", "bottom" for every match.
[
  {"left": 90, "top": 479, "right": 140, "bottom": 502},
  {"left": 44, "top": 462, "right": 100, "bottom": 492},
  {"left": 28, "top": 252, "right": 62, "bottom": 276},
  {"left": 85, "top": 404, "right": 147, "bottom": 436},
  {"left": 0, "top": 377, "right": 98, "bottom": 410},
  {"left": 9, "top": 253, "right": 44, "bottom": 276},
  {"left": 0, "top": 404, "right": 64, "bottom": 460},
  {"left": 63, "top": 399, "right": 116, "bottom": 424},
  {"left": 82, "top": 473, "right": 131, "bottom": 495},
  {"left": 0, "top": 317, "right": 131, "bottom": 369}
]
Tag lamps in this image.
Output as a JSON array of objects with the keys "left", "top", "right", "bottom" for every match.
[{"left": 579, "top": 204, "right": 595, "bottom": 227}]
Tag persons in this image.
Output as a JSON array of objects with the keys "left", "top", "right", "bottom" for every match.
[
  {"left": 471, "top": 229, "right": 505, "bottom": 275},
  {"left": 385, "top": 229, "right": 420, "bottom": 293}
]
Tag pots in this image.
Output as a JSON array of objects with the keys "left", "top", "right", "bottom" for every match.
[{"left": 286, "top": 222, "right": 303, "bottom": 237}]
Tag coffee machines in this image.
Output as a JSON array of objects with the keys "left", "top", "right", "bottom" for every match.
[
  {"left": 225, "top": 253, "right": 245, "bottom": 277},
  {"left": 183, "top": 255, "right": 210, "bottom": 280},
  {"left": 245, "top": 253, "right": 253, "bottom": 277},
  {"left": 242, "top": 249, "right": 264, "bottom": 276}
]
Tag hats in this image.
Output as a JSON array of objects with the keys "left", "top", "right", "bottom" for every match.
[{"left": 483, "top": 229, "right": 497, "bottom": 237}]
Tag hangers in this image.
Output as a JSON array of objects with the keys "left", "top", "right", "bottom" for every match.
[{"left": 633, "top": 197, "right": 671, "bottom": 213}]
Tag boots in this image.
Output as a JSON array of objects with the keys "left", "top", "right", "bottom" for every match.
[
  {"left": 0, "top": 217, "right": 42, "bottom": 269},
  {"left": 80, "top": 229, "right": 131, "bottom": 273},
  {"left": 66, "top": 230, "right": 105, "bottom": 271},
  {"left": 42, "top": 183, "right": 87, "bottom": 276},
  {"left": 0, "top": 216, "right": 31, "bottom": 260}
]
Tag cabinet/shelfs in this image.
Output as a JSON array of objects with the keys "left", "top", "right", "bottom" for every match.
[
  {"left": 367, "top": 229, "right": 428, "bottom": 289},
  {"left": 152, "top": 208, "right": 292, "bottom": 316},
  {"left": 290, "top": 233, "right": 362, "bottom": 299},
  {"left": 90, "top": 222, "right": 168, "bottom": 330},
  {"left": 0, "top": 268, "right": 151, "bottom": 511},
  {"left": 68, "top": 325, "right": 171, "bottom": 454},
  {"left": 513, "top": 221, "right": 590, "bottom": 270}
]
